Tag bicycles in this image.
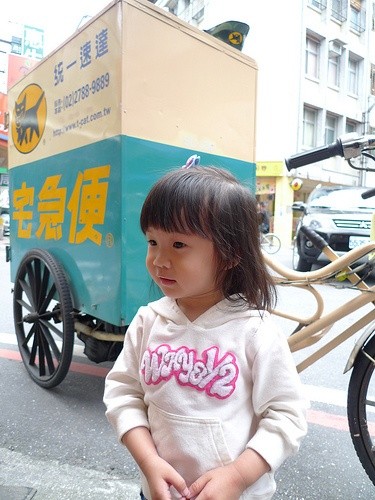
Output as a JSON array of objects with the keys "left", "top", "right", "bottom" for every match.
[{"left": 259, "top": 227, "right": 281, "bottom": 258}]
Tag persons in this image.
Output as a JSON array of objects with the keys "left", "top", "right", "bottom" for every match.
[
  {"left": 102, "top": 168, "right": 308, "bottom": 500},
  {"left": 256, "top": 201, "right": 268, "bottom": 232}
]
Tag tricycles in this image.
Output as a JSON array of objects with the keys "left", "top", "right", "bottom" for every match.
[{"left": 6, "top": 1, "right": 375, "bottom": 487}]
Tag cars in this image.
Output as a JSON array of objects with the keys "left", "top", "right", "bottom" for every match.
[{"left": 294, "top": 182, "right": 375, "bottom": 282}]
